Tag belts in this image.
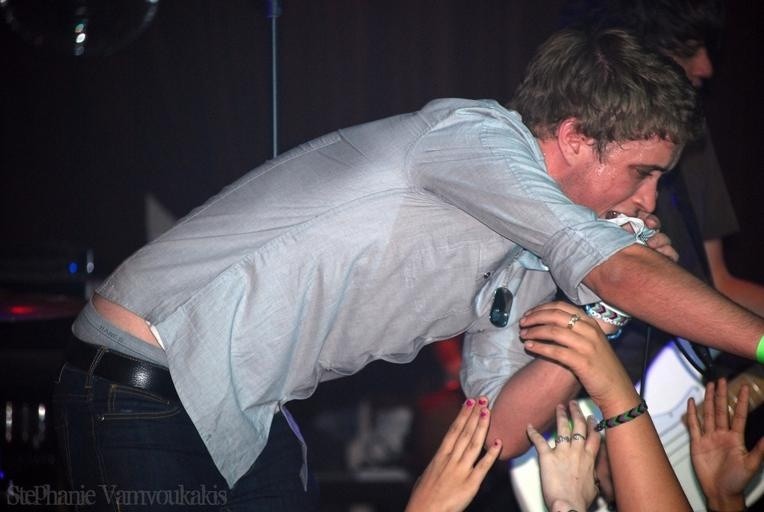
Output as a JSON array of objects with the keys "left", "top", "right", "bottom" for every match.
[{"left": 63, "top": 328, "right": 182, "bottom": 403}]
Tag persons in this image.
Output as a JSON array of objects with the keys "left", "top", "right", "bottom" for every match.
[
  {"left": 502, "top": 0, "right": 764, "bottom": 510},
  {"left": 51, "top": 21, "right": 764, "bottom": 510},
  {"left": 401, "top": 294, "right": 764, "bottom": 510}
]
